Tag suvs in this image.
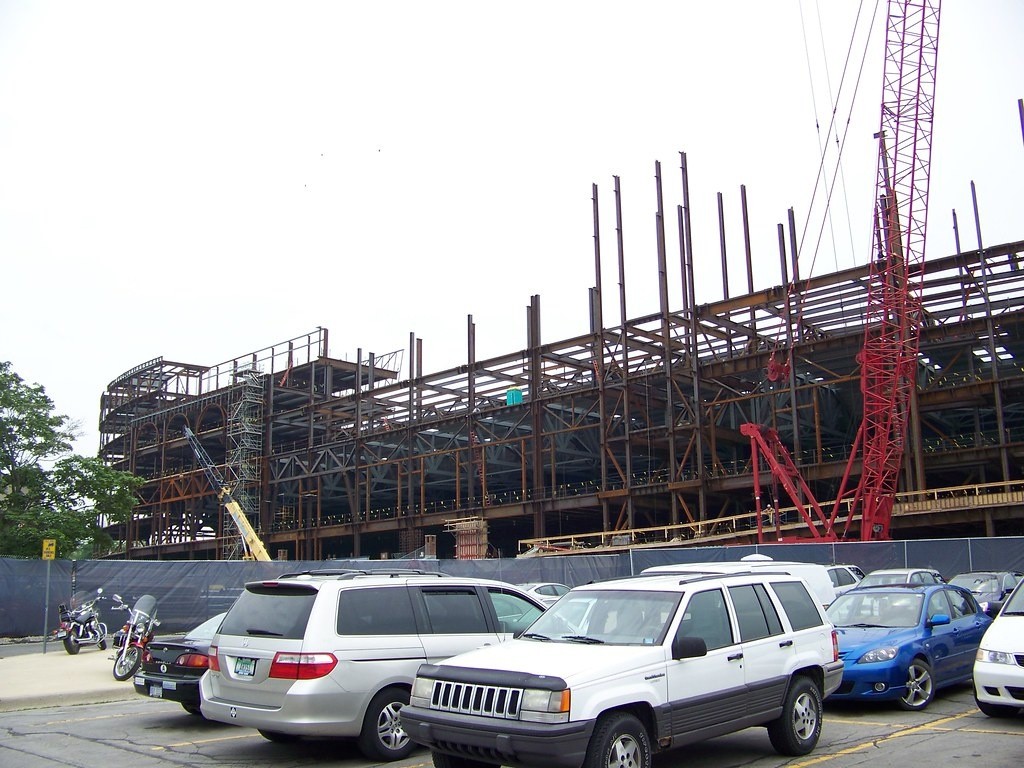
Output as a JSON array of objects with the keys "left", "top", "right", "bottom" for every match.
[
  {"left": 398, "top": 570, "right": 846, "bottom": 768},
  {"left": 199, "top": 567, "right": 588, "bottom": 761},
  {"left": 486, "top": 553, "right": 866, "bottom": 635}
]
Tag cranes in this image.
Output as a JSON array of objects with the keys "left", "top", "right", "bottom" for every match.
[{"left": 722, "top": 0, "right": 947, "bottom": 548}]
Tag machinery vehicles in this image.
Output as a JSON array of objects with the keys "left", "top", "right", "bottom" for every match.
[{"left": 182, "top": 424, "right": 272, "bottom": 561}]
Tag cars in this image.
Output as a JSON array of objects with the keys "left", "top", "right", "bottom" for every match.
[
  {"left": 834, "top": 569, "right": 946, "bottom": 619},
  {"left": 942, "top": 568, "right": 1024, "bottom": 619},
  {"left": 823, "top": 581, "right": 994, "bottom": 713},
  {"left": 971, "top": 576, "right": 1024, "bottom": 719},
  {"left": 132, "top": 610, "right": 229, "bottom": 718}
]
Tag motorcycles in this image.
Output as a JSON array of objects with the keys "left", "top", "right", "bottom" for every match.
[
  {"left": 110, "top": 594, "right": 161, "bottom": 682},
  {"left": 52, "top": 588, "right": 108, "bottom": 655}
]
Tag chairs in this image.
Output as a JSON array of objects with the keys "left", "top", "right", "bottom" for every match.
[
  {"left": 687, "top": 600, "right": 727, "bottom": 644},
  {"left": 613, "top": 602, "right": 643, "bottom": 637}
]
